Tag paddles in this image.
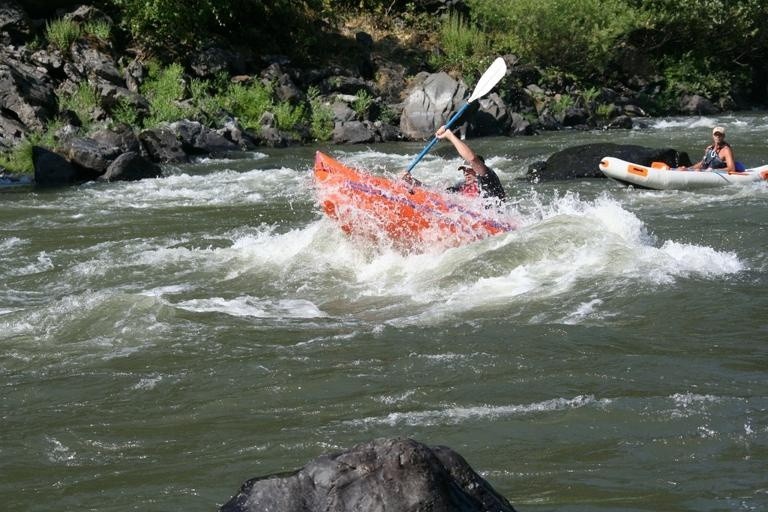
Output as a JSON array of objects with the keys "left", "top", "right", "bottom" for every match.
[
  {"left": 398, "top": 57, "right": 508, "bottom": 180},
  {"left": 650, "top": 161, "right": 753, "bottom": 176}
]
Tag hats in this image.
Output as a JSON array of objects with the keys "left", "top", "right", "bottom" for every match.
[
  {"left": 457, "top": 155, "right": 484, "bottom": 171},
  {"left": 713, "top": 127, "right": 725, "bottom": 135}
]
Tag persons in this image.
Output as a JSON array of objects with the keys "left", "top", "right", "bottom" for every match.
[
  {"left": 402, "top": 125, "right": 506, "bottom": 217},
  {"left": 677, "top": 126, "right": 735, "bottom": 170}
]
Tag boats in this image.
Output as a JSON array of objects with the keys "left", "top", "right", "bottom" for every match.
[
  {"left": 315, "top": 150, "right": 513, "bottom": 253},
  {"left": 599, "top": 156, "right": 768, "bottom": 192}
]
